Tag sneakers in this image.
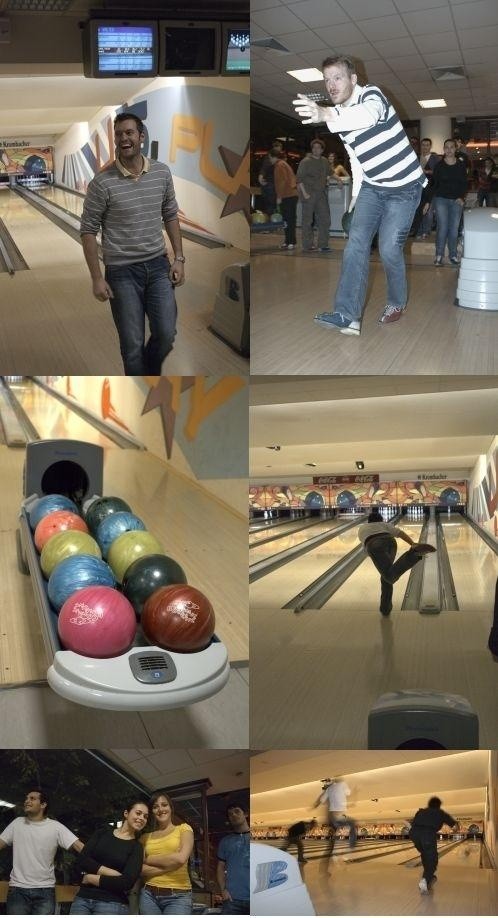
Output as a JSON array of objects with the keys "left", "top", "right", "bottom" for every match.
[
  {"left": 314, "top": 312, "right": 361, "bottom": 334},
  {"left": 410, "top": 542, "right": 436, "bottom": 557},
  {"left": 282, "top": 230, "right": 461, "bottom": 267},
  {"left": 378, "top": 303, "right": 407, "bottom": 323}
]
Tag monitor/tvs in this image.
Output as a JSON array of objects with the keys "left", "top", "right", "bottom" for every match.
[
  {"left": 225, "top": 24, "right": 252, "bottom": 77},
  {"left": 84, "top": 19, "right": 160, "bottom": 78},
  {"left": 160, "top": 23, "right": 224, "bottom": 78}
]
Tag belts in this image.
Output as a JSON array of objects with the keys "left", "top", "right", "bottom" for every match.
[{"left": 141, "top": 883, "right": 192, "bottom": 898}]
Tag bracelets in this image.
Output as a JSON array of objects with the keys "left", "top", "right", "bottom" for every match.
[{"left": 173, "top": 256, "right": 185, "bottom": 263}]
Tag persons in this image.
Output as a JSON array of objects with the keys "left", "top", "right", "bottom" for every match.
[
  {"left": 0, "top": 790, "right": 85, "bottom": 914},
  {"left": 69, "top": 798, "right": 150, "bottom": 915},
  {"left": 258, "top": 135, "right": 496, "bottom": 267},
  {"left": 291, "top": 54, "right": 429, "bottom": 336},
  {"left": 79, "top": 113, "right": 184, "bottom": 376},
  {"left": 407, "top": 797, "right": 460, "bottom": 895},
  {"left": 314, "top": 776, "right": 357, "bottom": 878},
  {"left": 280, "top": 820, "right": 317, "bottom": 863},
  {"left": 358, "top": 507, "right": 436, "bottom": 616},
  {"left": 215, "top": 802, "right": 250, "bottom": 915},
  {"left": 138, "top": 791, "right": 194, "bottom": 915}
]
